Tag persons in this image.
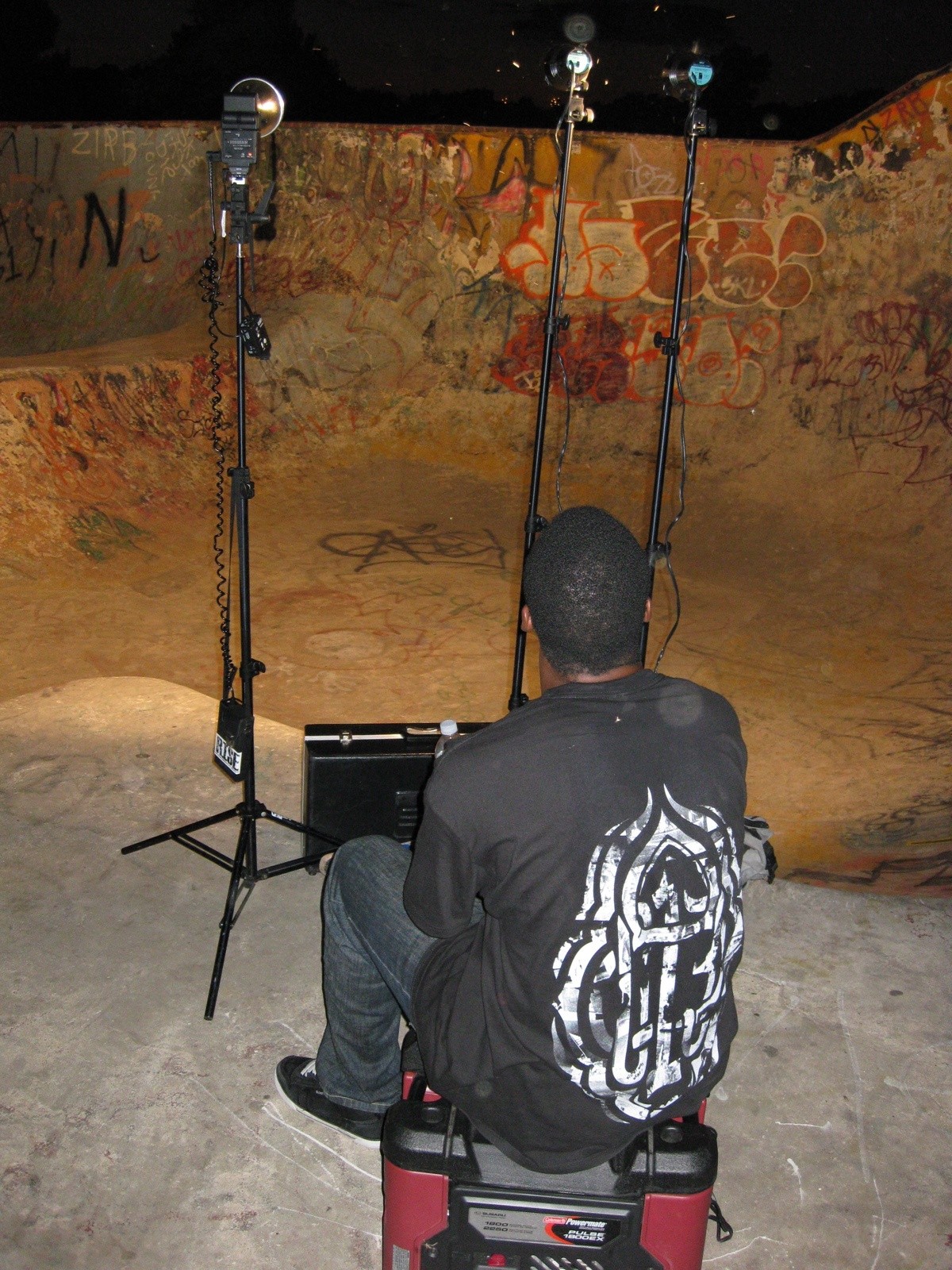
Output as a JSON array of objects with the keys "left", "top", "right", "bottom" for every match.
[{"left": 277, "top": 505, "right": 749, "bottom": 1175}]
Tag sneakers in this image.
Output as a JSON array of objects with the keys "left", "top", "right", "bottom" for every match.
[{"left": 274, "top": 1054, "right": 385, "bottom": 1147}]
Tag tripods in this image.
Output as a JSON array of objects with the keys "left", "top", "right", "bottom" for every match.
[{"left": 123, "top": 176, "right": 352, "bottom": 1018}]
